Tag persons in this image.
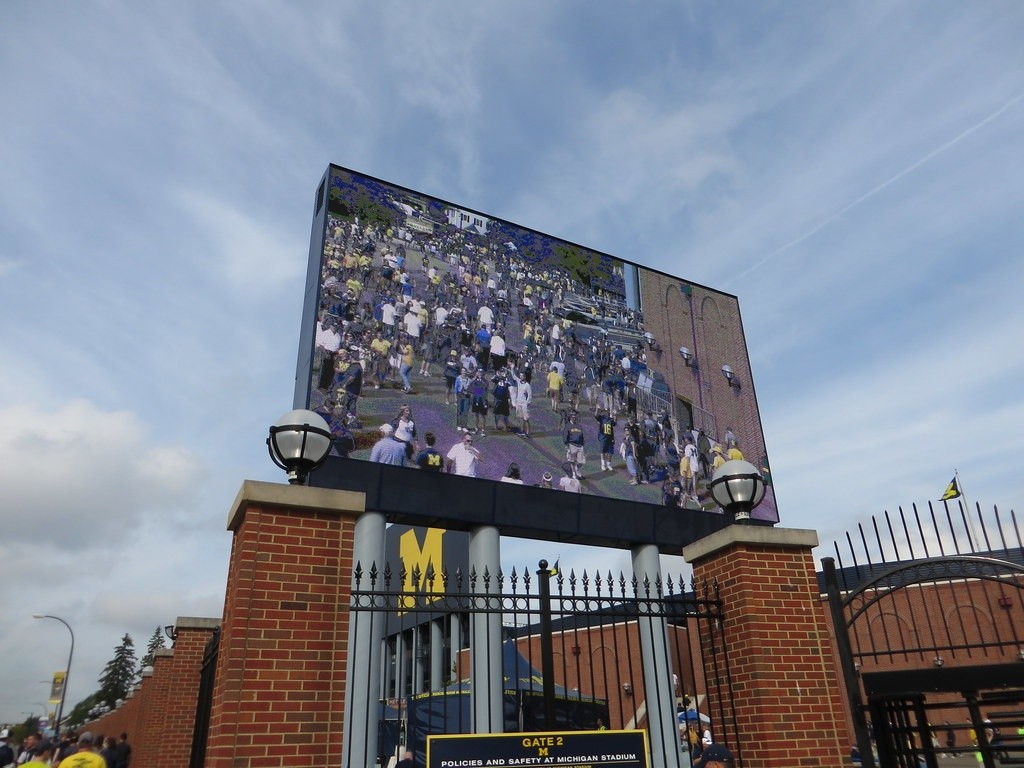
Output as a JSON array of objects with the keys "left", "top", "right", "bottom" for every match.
[
  {"left": 869, "top": 716, "right": 1002, "bottom": 768},
  {"left": 315, "top": 218, "right": 744, "bottom": 511},
  {"left": 597, "top": 716, "right": 610, "bottom": 730},
  {"left": 0, "top": 723, "right": 132, "bottom": 768},
  {"left": 687, "top": 724, "right": 736, "bottom": 768},
  {"left": 676, "top": 694, "right": 692, "bottom": 712},
  {"left": 393, "top": 750, "right": 423, "bottom": 768}
]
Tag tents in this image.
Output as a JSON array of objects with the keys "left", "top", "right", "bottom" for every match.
[{"left": 407, "top": 637, "right": 610, "bottom": 768}]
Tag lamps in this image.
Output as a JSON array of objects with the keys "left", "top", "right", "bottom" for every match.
[
  {"left": 572, "top": 687, "right": 579, "bottom": 692},
  {"left": 266, "top": 408, "right": 338, "bottom": 486},
  {"left": 706, "top": 459, "right": 767, "bottom": 524},
  {"left": 644, "top": 330, "right": 740, "bottom": 390},
  {"left": 623, "top": 683, "right": 633, "bottom": 696},
  {"left": 854, "top": 644, "right": 1024, "bottom": 678},
  {"left": 164, "top": 620, "right": 176, "bottom": 649}
]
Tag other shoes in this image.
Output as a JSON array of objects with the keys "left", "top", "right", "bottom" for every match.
[
  {"left": 951, "top": 756, "right": 956, "bottom": 759},
  {"left": 496, "top": 425, "right": 504, "bottom": 430},
  {"left": 629, "top": 477, "right": 639, "bottom": 485},
  {"left": 457, "top": 427, "right": 461, "bottom": 431},
  {"left": 942, "top": 753, "right": 947, "bottom": 759},
  {"left": 463, "top": 428, "right": 468, "bottom": 432},
  {"left": 552, "top": 408, "right": 558, "bottom": 413},
  {"left": 516, "top": 431, "right": 526, "bottom": 436},
  {"left": 572, "top": 475, "right": 577, "bottom": 480},
  {"left": 472, "top": 428, "right": 479, "bottom": 434},
  {"left": 607, "top": 465, "right": 613, "bottom": 471},
  {"left": 481, "top": 430, "right": 486, "bottom": 437},
  {"left": 374, "top": 384, "right": 384, "bottom": 390},
  {"left": 506, "top": 428, "right": 513, "bottom": 432},
  {"left": 403, "top": 386, "right": 413, "bottom": 393},
  {"left": 690, "top": 497, "right": 698, "bottom": 502},
  {"left": 420, "top": 370, "right": 424, "bottom": 374},
  {"left": 576, "top": 470, "right": 582, "bottom": 478},
  {"left": 642, "top": 480, "right": 648, "bottom": 484},
  {"left": 601, "top": 465, "right": 606, "bottom": 471},
  {"left": 424, "top": 371, "right": 430, "bottom": 376},
  {"left": 958, "top": 755, "right": 964, "bottom": 758}
]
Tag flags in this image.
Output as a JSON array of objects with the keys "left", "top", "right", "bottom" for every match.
[{"left": 938, "top": 474, "right": 962, "bottom": 502}]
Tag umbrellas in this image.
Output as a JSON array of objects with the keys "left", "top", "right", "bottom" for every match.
[{"left": 678, "top": 708, "right": 712, "bottom": 730}]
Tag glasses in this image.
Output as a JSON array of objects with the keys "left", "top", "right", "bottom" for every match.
[{"left": 465, "top": 438, "right": 473, "bottom": 443}]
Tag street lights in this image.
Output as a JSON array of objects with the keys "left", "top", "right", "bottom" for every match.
[{"left": 22, "top": 613, "right": 75, "bottom": 749}]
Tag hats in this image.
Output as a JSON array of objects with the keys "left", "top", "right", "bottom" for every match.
[
  {"left": 451, "top": 350, "right": 457, "bottom": 356},
  {"left": 380, "top": 423, "right": 393, "bottom": 432},
  {"left": 692, "top": 744, "right": 733, "bottom": 767},
  {"left": 712, "top": 446, "right": 721, "bottom": 453},
  {"left": 730, "top": 439, "right": 738, "bottom": 447},
  {"left": 77, "top": 732, "right": 94, "bottom": 747},
  {"left": 481, "top": 324, "right": 486, "bottom": 329},
  {"left": 0, "top": 729, "right": 13, "bottom": 739},
  {"left": 668, "top": 467, "right": 677, "bottom": 476},
  {"left": 604, "top": 407, "right": 611, "bottom": 414}
]
